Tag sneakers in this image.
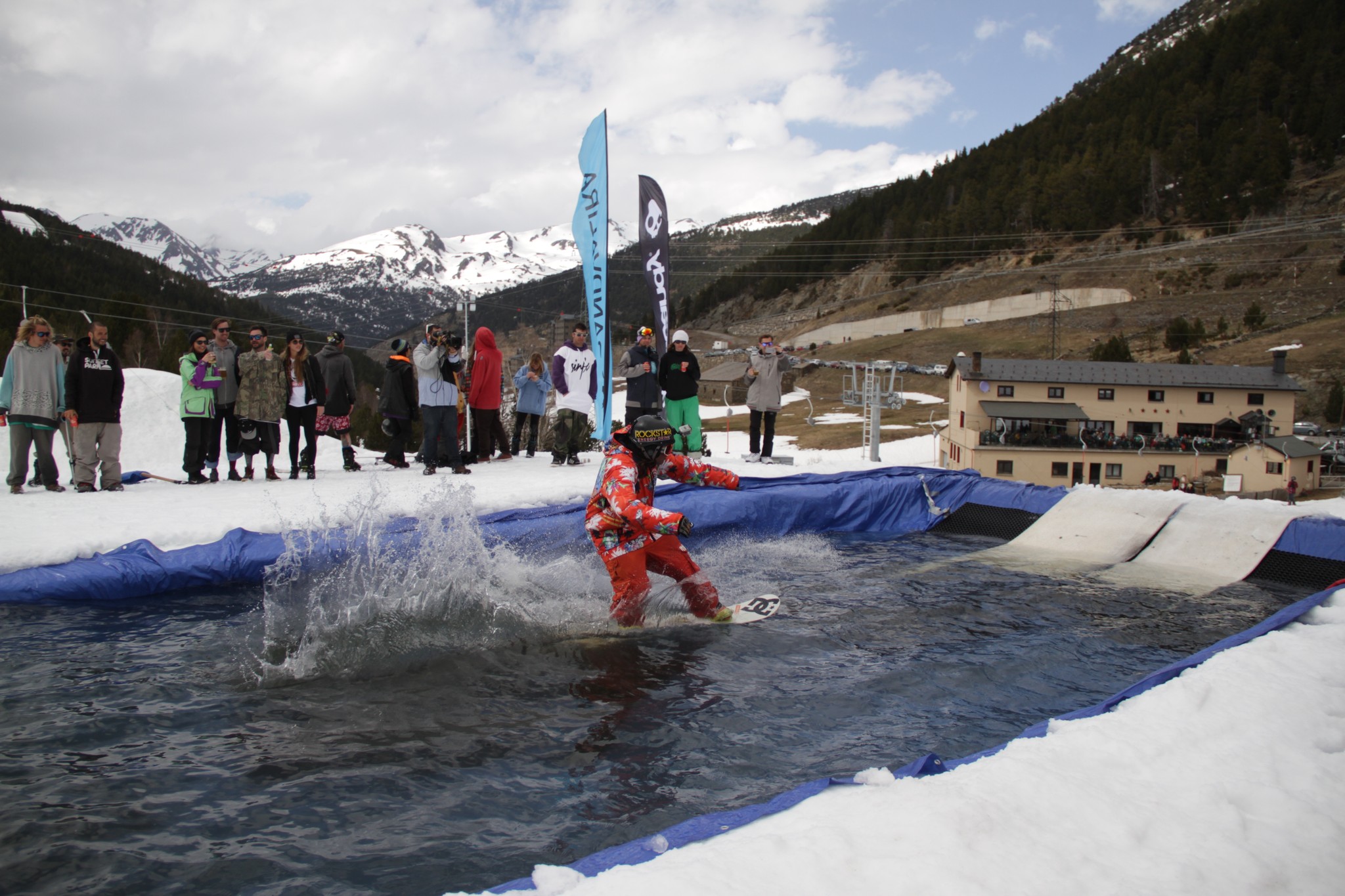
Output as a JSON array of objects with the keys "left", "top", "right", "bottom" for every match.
[
  {"left": 760, "top": 456, "right": 774, "bottom": 464},
  {"left": 745, "top": 452, "right": 761, "bottom": 462}
]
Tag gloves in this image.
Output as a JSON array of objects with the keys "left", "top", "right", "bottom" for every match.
[{"left": 678, "top": 517, "right": 693, "bottom": 538}]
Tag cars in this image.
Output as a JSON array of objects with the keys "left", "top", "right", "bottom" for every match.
[
  {"left": 1293, "top": 421, "right": 1322, "bottom": 436},
  {"left": 1320, "top": 440, "right": 1345, "bottom": 472},
  {"left": 788, "top": 356, "right": 948, "bottom": 377},
  {"left": 704, "top": 346, "right": 759, "bottom": 356},
  {"left": 1325, "top": 425, "right": 1345, "bottom": 438}
]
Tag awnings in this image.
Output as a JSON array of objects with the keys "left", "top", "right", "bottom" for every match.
[{"left": 978, "top": 400, "right": 1090, "bottom": 420}]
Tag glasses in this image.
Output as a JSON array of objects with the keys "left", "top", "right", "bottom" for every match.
[
  {"left": 639, "top": 329, "right": 653, "bottom": 337},
  {"left": 289, "top": 340, "right": 304, "bottom": 345},
  {"left": 574, "top": 332, "right": 588, "bottom": 338},
  {"left": 761, "top": 342, "right": 773, "bottom": 346},
  {"left": 57, "top": 343, "right": 72, "bottom": 348},
  {"left": 216, "top": 327, "right": 231, "bottom": 333},
  {"left": 33, "top": 332, "right": 50, "bottom": 337},
  {"left": 675, "top": 340, "right": 687, "bottom": 344},
  {"left": 196, "top": 340, "right": 208, "bottom": 345},
  {"left": 249, "top": 335, "right": 264, "bottom": 340}
]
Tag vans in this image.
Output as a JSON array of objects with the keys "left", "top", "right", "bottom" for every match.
[{"left": 964, "top": 318, "right": 981, "bottom": 325}]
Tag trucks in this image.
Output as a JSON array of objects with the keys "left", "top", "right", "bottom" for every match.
[{"left": 712, "top": 341, "right": 728, "bottom": 351}]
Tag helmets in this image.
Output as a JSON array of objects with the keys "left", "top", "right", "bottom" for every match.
[{"left": 628, "top": 413, "right": 676, "bottom": 448}]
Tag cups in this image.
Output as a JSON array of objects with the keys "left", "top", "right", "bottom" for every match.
[
  {"left": 754, "top": 366, "right": 759, "bottom": 377},
  {"left": 218, "top": 369, "right": 227, "bottom": 379},
  {"left": 208, "top": 352, "right": 216, "bottom": 363}
]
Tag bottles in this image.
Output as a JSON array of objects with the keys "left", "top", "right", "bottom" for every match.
[{"left": 265, "top": 343, "right": 273, "bottom": 361}]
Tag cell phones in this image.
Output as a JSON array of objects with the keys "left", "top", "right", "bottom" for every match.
[{"left": 766, "top": 347, "right": 776, "bottom": 353}]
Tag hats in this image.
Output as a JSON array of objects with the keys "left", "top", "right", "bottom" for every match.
[
  {"left": 636, "top": 327, "right": 652, "bottom": 343},
  {"left": 327, "top": 331, "right": 344, "bottom": 346},
  {"left": 286, "top": 330, "right": 303, "bottom": 343},
  {"left": 671, "top": 330, "right": 689, "bottom": 346},
  {"left": 188, "top": 330, "right": 207, "bottom": 346},
  {"left": 391, "top": 339, "right": 409, "bottom": 354},
  {"left": 52, "top": 333, "right": 75, "bottom": 343}
]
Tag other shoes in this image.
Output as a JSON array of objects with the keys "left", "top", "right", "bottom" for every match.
[
  {"left": 187, "top": 440, "right": 582, "bottom": 485},
  {"left": 77, "top": 482, "right": 92, "bottom": 492},
  {"left": 46, "top": 485, "right": 66, "bottom": 493},
  {"left": 28, "top": 476, "right": 42, "bottom": 487},
  {"left": 10, "top": 483, "right": 23, "bottom": 494},
  {"left": 107, "top": 482, "right": 124, "bottom": 491}
]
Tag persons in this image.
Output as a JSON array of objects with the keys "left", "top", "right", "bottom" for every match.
[
  {"left": 550, "top": 323, "right": 598, "bottom": 467},
  {"left": 178, "top": 329, "right": 222, "bottom": 484},
  {"left": 206, "top": 317, "right": 243, "bottom": 482},
  {"left": 585, "top": 414, "right": 741, "bottom": 632},
  {"left": 28, "top": 333, "right": 76, "bottom": 488},
  {"left": 413, "top": 324, "right": 513, "bottom": 475},
  {"left": 298, "top": 331, "right": 361, "bottom": 472},
  {"left": 280, "top": 328, "right": 325, "bottom": 479},
  {"left": 510, "top": 352, "right": 553, "bottom": 458},
  {"left": 0, "top": 314, "right": 67, "bottom": 494},
  {"left": 379, "top": 339, "right": 420, "bottom": 468},
  {"left": 234, "top": 325, "right": 288, "bottom": 481},
  {"left": 616, "top": 326, "right": 702, "bottom": 462},
  {"left": 992, "top": 422, "right": 1254, "bottom": 493},
  {"left": 62, "top": 321, "right": 124, "bottom": 491},
  {"left": 743, "top": 335, "right": 792, "bottom": 464},
  {"left": 1288, "top": 476, "right": 1298, "bottom": 505}
]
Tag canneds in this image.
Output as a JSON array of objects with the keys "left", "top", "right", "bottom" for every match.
[
  {"left": 0, "top": 415, "right": 6, "bottom": 426},
  {"left": 680, "top": 362, "right": 686, "bottom": 372},
  {"left": 70, "top": 415, "right": 78, "bottom": 427},
  {"left": 644, "top": 362, "right": 651, "bottom": 372}
]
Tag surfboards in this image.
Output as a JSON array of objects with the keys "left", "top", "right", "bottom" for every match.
[{"left": 560, "top": 594, "right": 781, "bottom": 647}]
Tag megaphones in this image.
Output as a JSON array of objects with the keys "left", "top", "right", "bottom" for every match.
[
  {"left": 1268, "top": 409, "right": 1276, "bottom": 417},
  {"left": 1256, "top": 408, "right": 1264, "bottom": 416}
]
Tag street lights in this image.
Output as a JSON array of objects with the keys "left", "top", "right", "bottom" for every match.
[{"left": 457, "top": 294, "right": 476, "bottom": 453}]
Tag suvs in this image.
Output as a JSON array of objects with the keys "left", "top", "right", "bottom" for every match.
[{"left": 781, "top": 345, "right": 794, "bottom": 352}]
